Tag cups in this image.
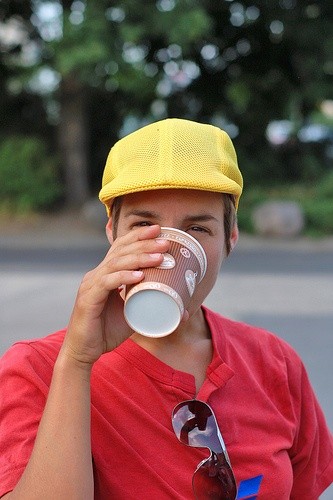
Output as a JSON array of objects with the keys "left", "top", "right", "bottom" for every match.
[{"left": 123, "top": 227, "right": 208, "bottom": 338}]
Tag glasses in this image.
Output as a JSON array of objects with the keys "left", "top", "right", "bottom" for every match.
[{"left": 172, "top": 400, "right": 238, "bottom": 500}]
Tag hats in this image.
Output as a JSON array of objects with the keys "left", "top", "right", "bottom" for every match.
[{"left": 98, "top": 118, "right": 243, "bottom": 220}]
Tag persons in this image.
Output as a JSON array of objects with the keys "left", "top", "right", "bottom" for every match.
[{"left": 0, "top": 119, "right": 333, "bottom": 500}]
